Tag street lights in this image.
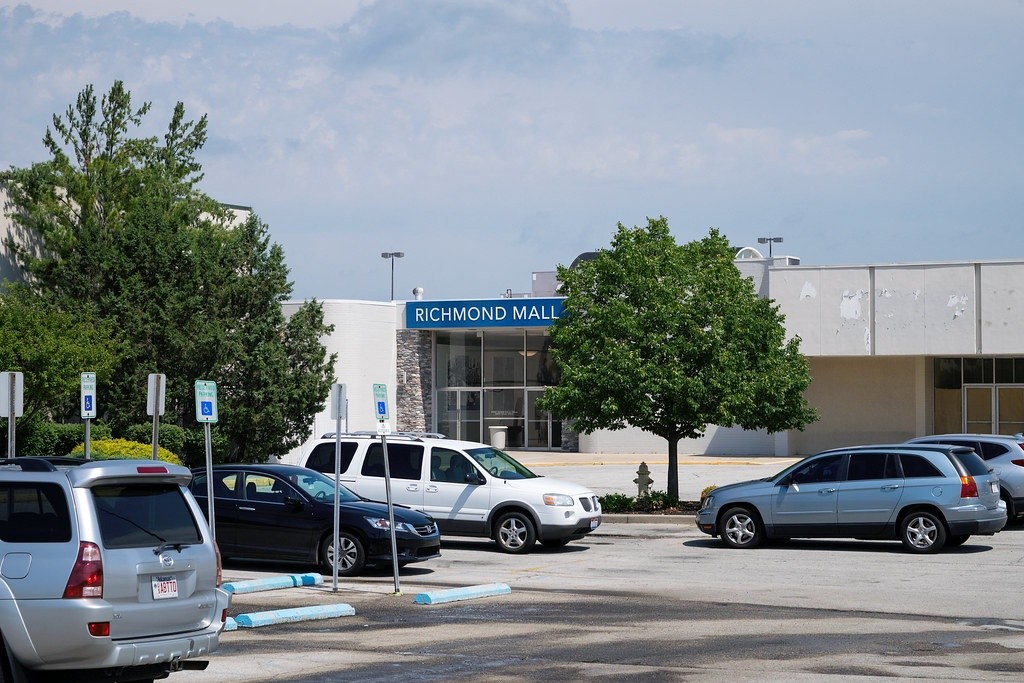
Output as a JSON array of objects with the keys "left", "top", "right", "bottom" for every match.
[
  {"left": 757, "top": 236, "right": 784, "bottom": 257},
  {"left": 382, "top": 251, "right": 405, "bottom": 301}
]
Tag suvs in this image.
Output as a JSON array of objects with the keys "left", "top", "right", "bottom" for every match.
[
  {"left": 695, "top": 442, "right": 1007, "bottom": 553},
  {"left": 0, "top": 454, "right": 229, "bottom": 677},
  {"left": 902, "top": 433, "right": 1024, "bottom": 515}
]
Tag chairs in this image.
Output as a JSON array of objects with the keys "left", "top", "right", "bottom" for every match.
[
  {"left": 446, "top": 455, "right": 470, "bottom": 482},
  {"left": 271, "top": 479, "right": 290, "bottom": 503},
  {"left": 430, "top": 456, "right": 448, "bottom": 481}
]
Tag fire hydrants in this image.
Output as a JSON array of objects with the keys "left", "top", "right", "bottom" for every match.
[{"left": 634, "top": 462, "right": 654, "bottom": 500}]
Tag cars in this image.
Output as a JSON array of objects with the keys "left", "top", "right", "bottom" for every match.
[{"left": 184, "top": 462, "right": 442, "bottom": 578}]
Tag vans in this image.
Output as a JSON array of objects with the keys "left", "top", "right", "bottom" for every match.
[{"left": 297, "top": 431, "right": 603, "bottom": 554}]
